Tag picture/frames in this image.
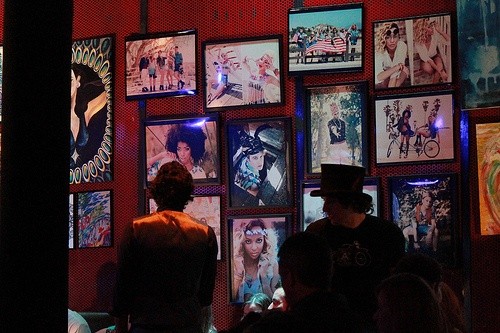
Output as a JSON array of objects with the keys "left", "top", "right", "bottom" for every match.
[
  {"left": 371, "top": 12, "right": 456, "bottom": 92},
  {"left": 225, "top": 116, "right": 294, "bottom": 209},
  {"left": 77, "top": 189, "right": 113, "bottom": 249},
  {"left": 142, "top": 112, "right": 220, "bottom": 189},
  {"left": 69, "top": 33, "right": 116, "bottom": 184},
  {"left": 372, "top": 88, "right": 457, "bottom": 166},
  {"left": 201, "top": 34, "right": 286, "bottom": 112},
  {"left": 287, "top": 2, "right": 365, "bottom": 76},
  {"left": 299, "top": 175, "right": 382, "bottom": 233},
  {"left": 69, "top": 192, "right": 76, "bottom": 250},
  {"left": 125, "top": 29, "right": 198, "bottom": 102},
  {"left": 386, "top": 172, "right": 459, "bottom": 268},
  {"left": 225, "top": 213, "right": 293, "bottom": 306},
  {"left": 304, "top": 80, "right": 370, "bottom": 177},
  {"left": 147, "top": 193, "right": 222, "bottom": 261}
]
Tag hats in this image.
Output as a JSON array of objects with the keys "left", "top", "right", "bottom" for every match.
[
  {"left": 309, "top": 163, "right": 372, "bottom": 202},
  {"left": 242, "top": 137, "right": 264, "bottom": 157},
  {"left": 402, "top": 109, "right": 412, "bottom": 119}
]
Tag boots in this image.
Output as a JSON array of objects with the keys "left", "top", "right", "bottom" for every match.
[{"left": 177, "top": 79, "right": 185, "bottom": 90}]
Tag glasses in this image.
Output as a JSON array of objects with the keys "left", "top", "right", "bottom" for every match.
[{"left": 386, "top": 29, "right": 398, "bottom": 36}]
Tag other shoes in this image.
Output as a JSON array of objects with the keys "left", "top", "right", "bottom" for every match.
[
  {"left": 141, "top": 87, "right": 149, "bottom": 92},
  {"left": 150, "top": 85, "right": 155, "bottom": 92},
  {"left": 414, "top": 140, "right": 422, "bottom": 147},
  {"left": 399, "top": 153, "right": 408, "bottom": 159},
  {"left": 168, "top": 84, "right": 174, "bottom": 89},
  {"left": 160, "top": 85, "right": 164, "bottom": 90}
]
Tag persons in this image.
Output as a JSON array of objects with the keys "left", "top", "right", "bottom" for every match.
[
  {"left": 326, "top": 101, "right": 349, "bottom": 165},
  {"left": 385, "top": 105, "right": 440, "bottom": 159},
  {"left": 69, "top": 67, "right": 106, "bottom": 171},
  {"left": 241, "top": 52, "right": 280, "bottom": 104},
  {"left": 413, "top": 18, "right": 451, "bottom": 83},
  {"left": 234, "top": 132, "right": 265, "bottom": 186},
  {"left": 148, "top": 125, "right": 207, "bottom": 181},
  {"left": 233, "top": 219, "right": 279, "bottom": 300},
  {"left": 114, "top": 160, "right": 218, "bottom": 333},
  {"left": 373, "top": 255, "right": 467, "bottom": 333},
  {"left": 208, "top": 48, "right": 239, "bottom": 105},
  {"left": 238, "top": 232, "right": 372, "bottom": 333},
  {"left": 375, "top": 23, "right": 410, "bottom": 88},
  {"left": 296, "top": 24, "right": 359, "bottom": 63},
  {"left": 139, "top": 46, "right": 185, "bottom": 92},
  {"left": 305, "top": 164, "right": 405, "bottom": 325},
  {"left": 403, "top": 191, "right": 438, "bottom": 251}
]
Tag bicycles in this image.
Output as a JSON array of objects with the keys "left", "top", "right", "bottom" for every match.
[{"left": 386, "top": 133, "right": 440, "bottom": 158}]
